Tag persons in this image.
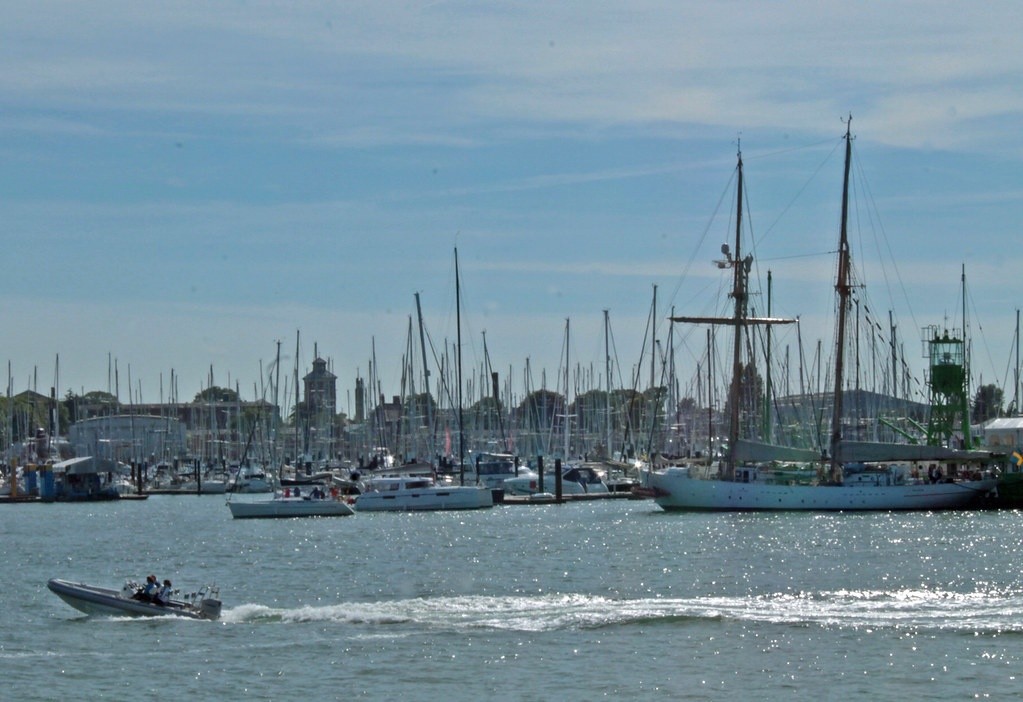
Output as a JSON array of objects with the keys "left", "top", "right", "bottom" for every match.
[
  {"left": 365, "top": 485, "right": 371, "bottom": 493},
  {"left": 911, "top": 465, "right": 943, "bottom": 484},
  {"left": 972, "top": 470, "right": 980, "bottom": 482},
  {"left": 285, "top": 485, "right": 339, "bottom": 499},
  {"left": 135, "top": 575, "right": 171, "bottom": 605}
]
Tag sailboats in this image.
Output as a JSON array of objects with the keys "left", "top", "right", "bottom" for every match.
[
  {"left": 1, "top": 244, "right": 1023, "bottom": 522},
  {"left": 626, "top": 109, "right": 1009, "bottom": 518}
]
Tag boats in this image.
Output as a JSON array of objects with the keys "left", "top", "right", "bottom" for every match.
[{"left": 46, "top": 577, "right": 224, "bottom": 621}]
